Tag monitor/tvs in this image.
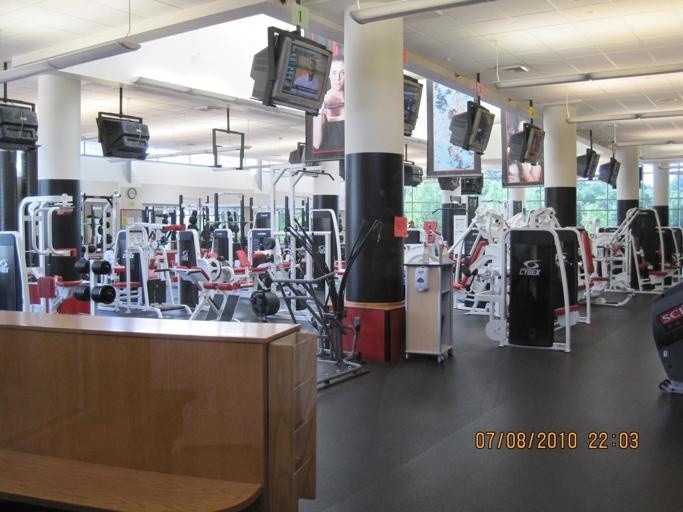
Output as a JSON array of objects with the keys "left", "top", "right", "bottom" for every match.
[
  {"left": 577, "top": 151, "right": 600, "bottom": 178},
  {"left": 448, "top": 108, "right": 495, "bottom": 152},
  {"left": 288, "top": 145, "right": 320, "bottom": 167},
  {"left": 461, "top": 173, "right": 483, "bottom": 194},
  {"left": 436, "top": 177, "right": 460, "bottom": 191},
  {"left": 403, "top": 163, "right": 423, "bottom": 187},
  {"left": 95, "top": 117, "right": 149, "bottom": 160},
  {"left": 1, "top": 106, "right": 39, "bottom": 150},
  {"left": 509, "top": 127, "right": 545, "bottom": 163},
  {"left": 250, "top": 36, "right": 332, "bottom": 109},
  {"left": 598, "top": 161, "right": 621, "bottom": 186}
]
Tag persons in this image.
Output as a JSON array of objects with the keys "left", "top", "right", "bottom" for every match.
[
  {"left": 291, "top": 53, "right": 320, "bottom": 98},
  {"left": 312, "top": 54, "right": 344, "bottom": 150},
  {"left": 507, "top": 119, "right": 542, "bottom": 182}
]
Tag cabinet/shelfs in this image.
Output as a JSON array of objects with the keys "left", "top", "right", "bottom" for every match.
[
  {"left": 0, "top": 309, "right": 317, "bottom": 512},
  {"left": 404, "top": 264, "right": 455, "bottom": 363}
]
